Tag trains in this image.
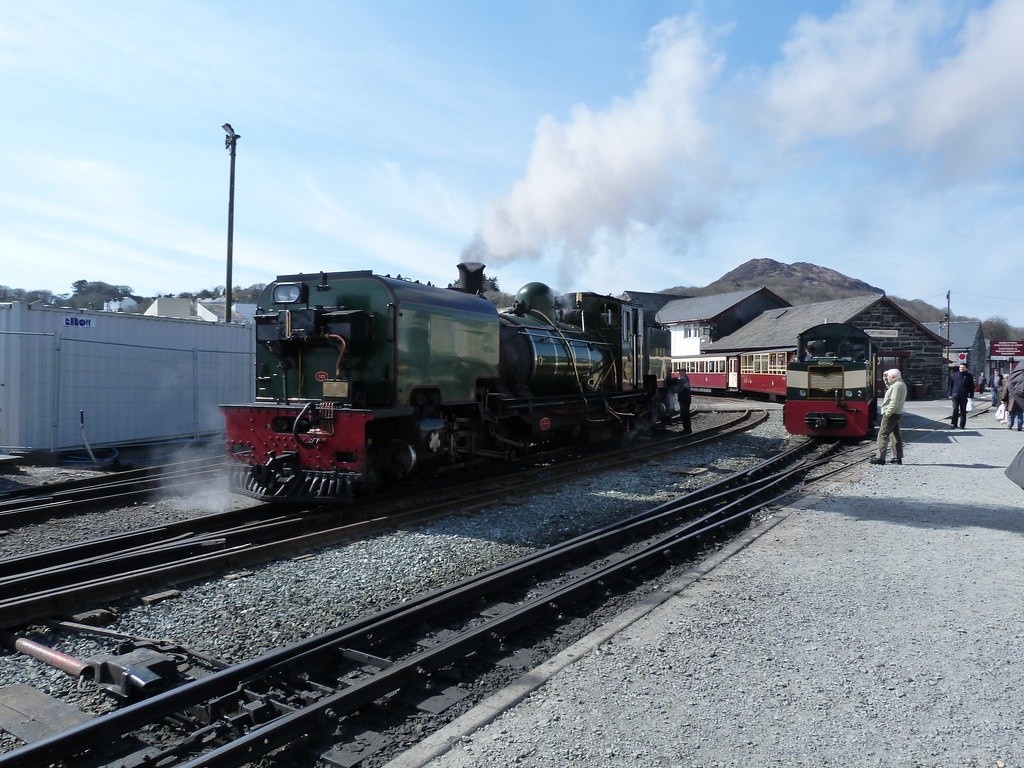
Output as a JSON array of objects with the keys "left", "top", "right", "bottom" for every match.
[
  {"left": 219, "top": 262, "right": 672, "bottom": 505},
  {"left": 622, "top": 318, "right": 877, "bottom": 437}
]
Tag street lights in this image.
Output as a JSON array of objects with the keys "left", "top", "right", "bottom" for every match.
[{"left": 222, "top": 123, "right": 241, "bottom": 322}]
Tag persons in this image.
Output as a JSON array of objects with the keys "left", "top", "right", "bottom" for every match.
[
  {"left": 670, "top": 368, "right": 692, "bottom": 434},
  {"left": 869, "top": 359, "right": 1024, "bottom": 489}
]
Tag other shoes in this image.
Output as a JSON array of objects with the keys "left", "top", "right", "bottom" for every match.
[
  {"left": 951, "top": 424, "right": 956, "bottom": 428},
  {"left": 1008, "top": 425, "right": 1011, "bottom": 429},
  {"left": 869, "top": 457, "right": 885, "bottom": 465},
  {"left": 961, "top": 426, "right": 964, "bottom": 429},
  {"left": 889, "top": 457, "right": 901, "bottom": 463},
  {"left": 1018, "top": 428, "right": 1021, "bottom": 431}
]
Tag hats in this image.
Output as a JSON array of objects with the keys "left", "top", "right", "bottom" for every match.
[{"left": 677, "top": 369, "right": 686, "bottom": 372}]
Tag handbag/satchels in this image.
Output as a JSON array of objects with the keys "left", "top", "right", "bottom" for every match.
[
  {"left": 966, "top": 397, "right": 976, "bottom": 413},
  {"left": 996, "top": 402, "right": 1009, "bottom": 425}
]
